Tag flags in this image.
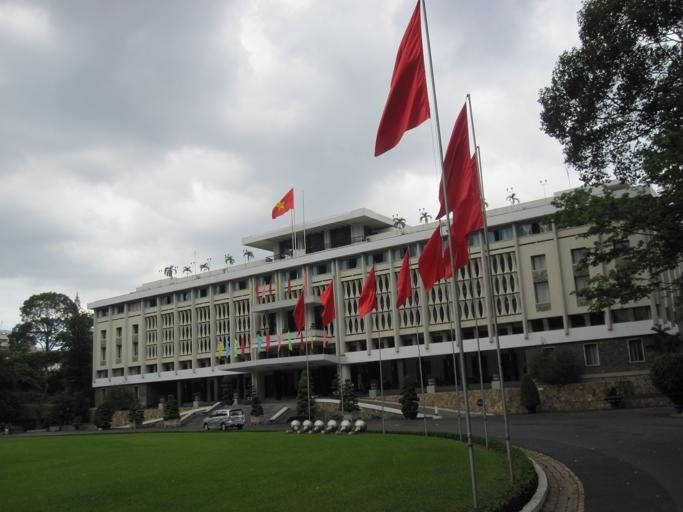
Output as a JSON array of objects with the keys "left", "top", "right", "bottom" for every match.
[
  {"left": 320, "top": 281, "right": 336, "bottom": 328},
  {"left": 358, "top": 266, "right": 379, "bottom": 320},
  {"left": 418, "top": 155, "right": 485, "bottom": 292},
  {"left": 272, "top": 188, "right": 293, "bottom": 219},
  {"left": 292, "top": 291, "right": 305, "bottom": 330},
  {"left": 374, "top": 0, "right": 431, "bottom": 157},
  {"left": 216, "top": 330, "right": 329, "bottom": 360},
  {"left": 395, "top": 247, "right": 412, "bottom": 310},
  {"left": 435, "top": 103, "right": 476, "bottom": 220},
  {"left": 254, "top": 279, "right": 302, "bottom": 294}
]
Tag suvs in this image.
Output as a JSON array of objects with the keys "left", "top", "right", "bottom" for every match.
[{"left": 202, "top": 409, "right": 246, "bottom": 431}]
